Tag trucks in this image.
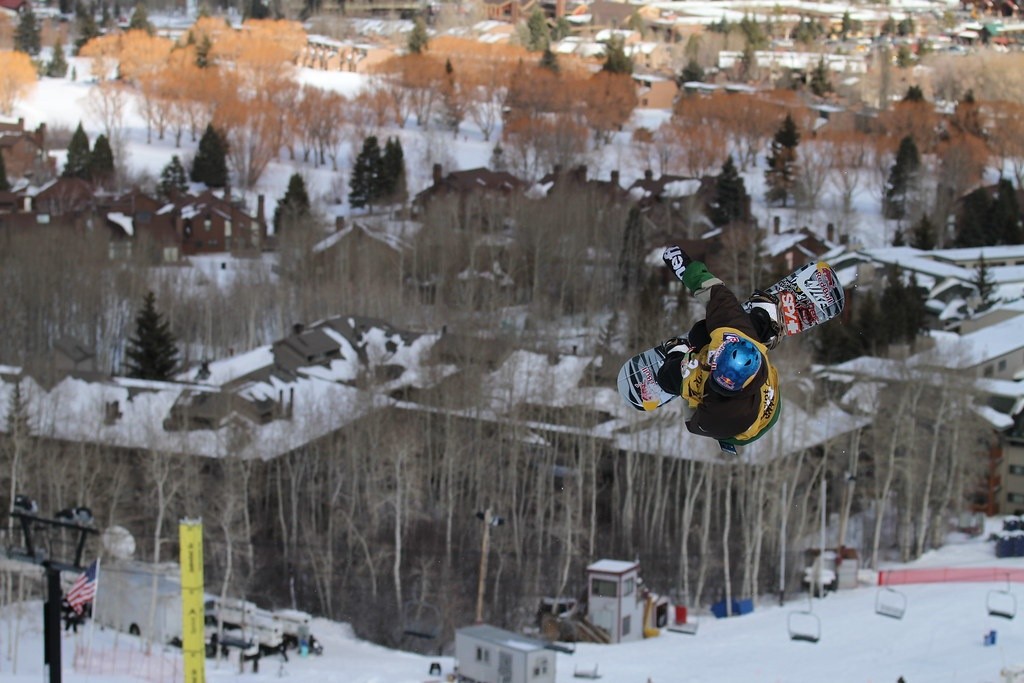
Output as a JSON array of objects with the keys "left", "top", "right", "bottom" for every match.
[{"left": 95, "top": 562, "right": 311, "bottom": 659}]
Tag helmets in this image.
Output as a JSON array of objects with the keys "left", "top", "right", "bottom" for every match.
[{"left": 708, "top": 337, "right": 761, "bottom": 392}]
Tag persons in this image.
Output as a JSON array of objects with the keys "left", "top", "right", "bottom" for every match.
[{"left": 656, "top": 244, "right": 782, "bottom": 447}]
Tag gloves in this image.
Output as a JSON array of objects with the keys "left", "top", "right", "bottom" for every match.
[
  {"left": 681, "top": 347, "right": 700, "bottom": 364},
  {"left": 663, "top": 245, "right": 694, "bottom": 284}
]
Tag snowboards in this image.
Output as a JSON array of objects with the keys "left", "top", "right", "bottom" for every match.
[{"left": 616, "top": 260, "right": 845, "bottom": 413}]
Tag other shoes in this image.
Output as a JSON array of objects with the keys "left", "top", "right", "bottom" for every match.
[
  {"left": 750, "top": 307, "right": 772, "bottom": 336},
  {"left": 664, "top": 338, "right": 690, "bottom": 354}
]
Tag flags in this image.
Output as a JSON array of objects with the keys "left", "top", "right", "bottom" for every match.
[{"left": 65, "top": 559, "right": 99, "bottom": 614}]
